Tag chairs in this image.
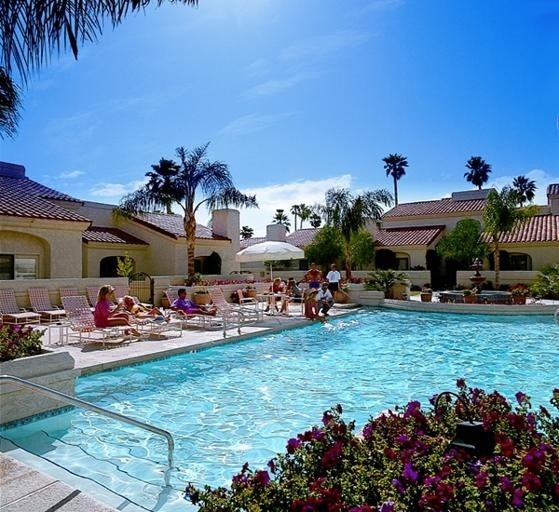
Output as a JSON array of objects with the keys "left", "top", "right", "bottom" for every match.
[
  {"left": 162, "top": 286, "right": 260, "bottom": 331},
  {"left": 27, "top": 289, "right": 67, "bottom": 322},
  {"left": 59, "top": 286, "right": 96, "bottom": 313},
  {"left": 282, "top": 283, "right": 306, "bottom": 315},
  {"left": 1, "top": 287, "right": 43, "bottom": 327},
  {"left": 86, "top": 285, "right": 154, "bottom": 309},
  {"left": 116, "top": 297, "right": 183, "bottom": 340},
  {"left": 60, "top": 295, "right": 134, "bottom": 347}
]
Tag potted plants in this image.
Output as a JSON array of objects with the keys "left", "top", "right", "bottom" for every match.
[{"left": 348, "top": 268, "right": 528, "bottom": 305}]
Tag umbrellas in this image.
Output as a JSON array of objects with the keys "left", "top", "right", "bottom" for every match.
[{"left": 235, "top": 241, "right": 305, "bottom": 293}]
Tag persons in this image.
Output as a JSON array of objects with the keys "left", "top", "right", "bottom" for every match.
[
  {"left": 304, "top": 262, "right": 322, "bottom": 289},
  {"left": 122, "top": 294, "right": 171, "bottom": 321},
  {"left": 316, "top": 281, "right": 335, "bottom": 316},
  {"left": 94, "top": 284, "right": 139, "bottom": 336},
  {"left": 326, "top": 263, "right": 341, "bottom": 294},
  {"left": 170, "top": 288, "right": 217, "bottom": 320},
  {"left": 304, "top": 287, "right": 325, "bottom": 322},
  {"left": 262, "top": 276, "right": 311, "bottom": 316}
]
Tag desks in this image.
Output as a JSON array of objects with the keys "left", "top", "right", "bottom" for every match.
[
  {"left": 254, "top": 293, "right": 285, "bottom": 316},
  {"left": 47, "top": 324, "right": 70, "bottom": 346}
]
